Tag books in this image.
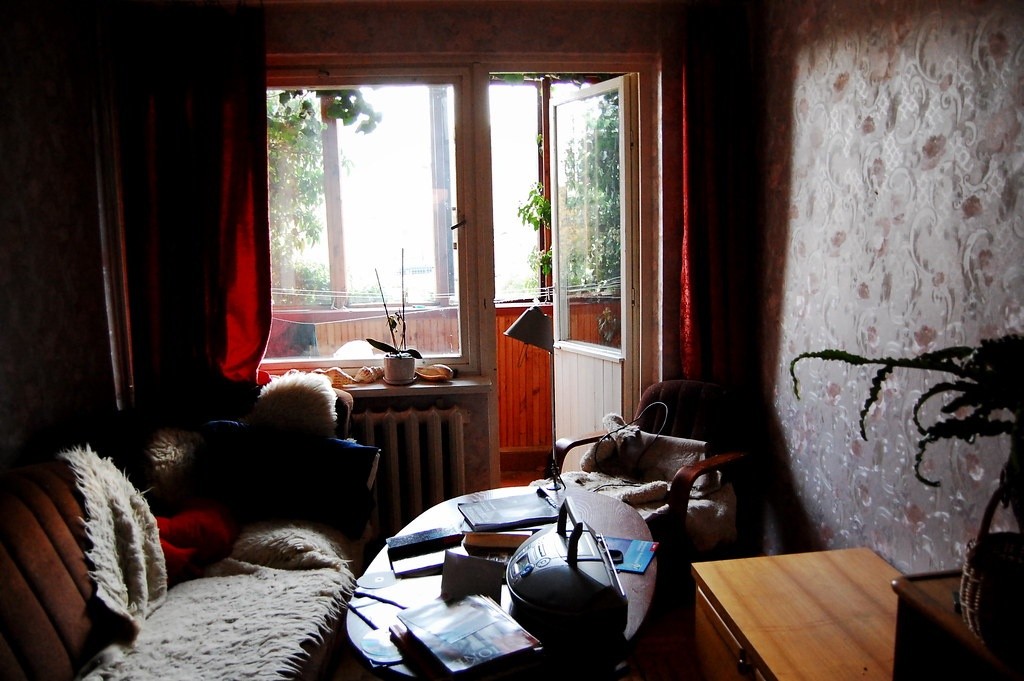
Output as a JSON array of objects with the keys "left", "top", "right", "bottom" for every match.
[
  {"left": 389, "top": 591, "right": 544, "bottom": 673},
  {"left": 462, "top": 529, "right": 533, "bottom": 548}
]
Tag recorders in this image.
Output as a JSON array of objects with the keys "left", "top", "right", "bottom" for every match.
[{"left": 505, "top": 496, "right": 628, "bottom": 631}]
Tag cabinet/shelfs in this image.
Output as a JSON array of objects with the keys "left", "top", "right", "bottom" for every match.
[{"left": 691, "top": 546, "right": 906, "bottom": 681}]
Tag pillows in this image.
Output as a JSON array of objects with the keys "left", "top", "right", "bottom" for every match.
[{"left": 169, "top": 418, "right": 382, "bottom": 542}]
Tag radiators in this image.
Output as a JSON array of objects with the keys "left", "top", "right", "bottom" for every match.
[{"left": 347, "top": 406, "right": 466, "bottom": 542}]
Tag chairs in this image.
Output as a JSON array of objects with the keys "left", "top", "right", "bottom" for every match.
[{"left": 547, "top": 377, "right": 746, "bottom": 574}]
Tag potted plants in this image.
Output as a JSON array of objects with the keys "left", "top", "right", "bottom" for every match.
[{"left": 365, "top": 247, "right": 423, "bottom": 385}]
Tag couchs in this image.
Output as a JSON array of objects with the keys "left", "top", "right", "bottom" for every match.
[{"left": 0, "top": 442, "right": 375, "bottom": 681}]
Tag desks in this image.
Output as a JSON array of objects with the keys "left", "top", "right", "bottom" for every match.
[{"left": 347, "top": 484, "right": 658, "bottom": 681}]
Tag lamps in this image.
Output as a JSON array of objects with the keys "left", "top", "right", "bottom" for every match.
[{"left": 502, "top": 305, "right": 559, "bottom": 486}]
